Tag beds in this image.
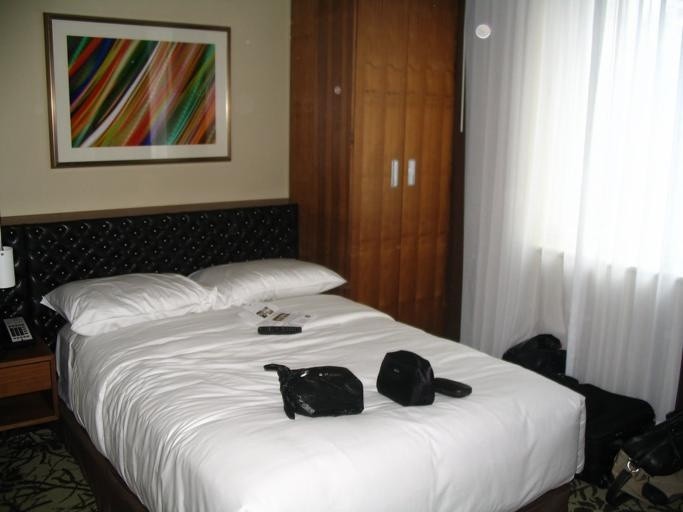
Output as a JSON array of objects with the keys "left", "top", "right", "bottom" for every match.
[{"left": 47, "top": 243, "right": 596, "bottom": 511}]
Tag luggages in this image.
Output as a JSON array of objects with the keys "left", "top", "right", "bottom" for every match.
[{"left": 543, "top": 370, "right": 656, "bottom": 490}]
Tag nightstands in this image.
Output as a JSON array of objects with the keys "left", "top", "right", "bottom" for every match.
[{"left": 1, "top": 318, "right": 62, "bottom": 436}]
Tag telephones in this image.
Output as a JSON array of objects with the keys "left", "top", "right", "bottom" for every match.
[{"left": 0, "top": 316, "right": 38, "bottom": 349}]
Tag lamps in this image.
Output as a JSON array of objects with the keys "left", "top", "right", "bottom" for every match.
[{"left": 1, "top": 240, "right": 18, "bottom": 292}]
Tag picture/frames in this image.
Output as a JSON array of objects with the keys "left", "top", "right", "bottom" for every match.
[{"left": 41, "top": 9, "right": 235, "bottom": 168}]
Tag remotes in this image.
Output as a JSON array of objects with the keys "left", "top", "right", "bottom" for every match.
[{"left": 258, "top": 326, "right": 302, "bottom": 335}]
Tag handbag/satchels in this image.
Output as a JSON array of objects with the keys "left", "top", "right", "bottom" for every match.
[
  {"left": 375, "top": 351, "right": 437, "bottom": 407},
  {"left": 278, "top": 365, "right": 364, "bottom": 418},
  {"left": 610, "top": 413, "right": 682, "bottom": 507},
  {"left": 504, "top": 333, "right": 566, "bottom": 373}
]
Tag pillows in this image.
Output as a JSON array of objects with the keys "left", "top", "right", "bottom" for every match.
[
  {"left": 187, "top": 254, "right": 352, "bottom": 313},
  {"left": 41, "top": 269, "right": 220, "bottom": 338}
]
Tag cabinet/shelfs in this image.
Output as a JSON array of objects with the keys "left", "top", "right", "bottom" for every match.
[{"left": 285, "top": 2, "right": 464, "bottom": 338}]
